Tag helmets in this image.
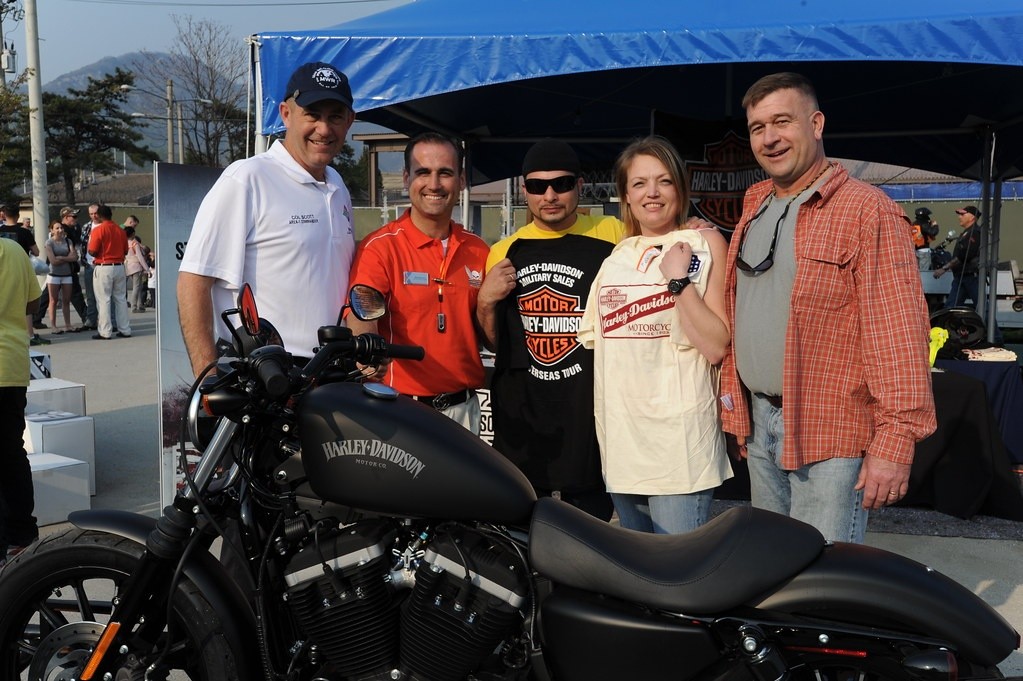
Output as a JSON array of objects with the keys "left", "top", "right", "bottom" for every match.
[{"left": 915, "top": 207, "right": 931, "bottom": 215}]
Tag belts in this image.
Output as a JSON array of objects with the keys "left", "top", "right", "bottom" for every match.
[
  {"left": 95, "top": 263, "right": 124, "bottom": 266},
  {"left": 755, "top": 392, "right": 782, "bottom": 409},
  {"left": 404, "top": 388, "right": 475, "bottom": 411}
]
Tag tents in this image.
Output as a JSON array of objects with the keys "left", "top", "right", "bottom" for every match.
[{"left": 246, "top": 0, "right": 1023, "bottom": 344}]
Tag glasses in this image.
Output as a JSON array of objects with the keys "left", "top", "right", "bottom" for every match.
[
  {"left": 736, "top": 205, "right": 788, "bottom": 274},
  {"left": 524, "top": 175, "right": 578, "bottom": 195}
]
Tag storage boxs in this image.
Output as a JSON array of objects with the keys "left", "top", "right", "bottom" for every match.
[{"left": 24, "top": 352, "right": 95, "bottom": 529}]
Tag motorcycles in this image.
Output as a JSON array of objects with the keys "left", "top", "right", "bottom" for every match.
[{"left": 0, "top": 280, "right": 1023, "bottom": 681}]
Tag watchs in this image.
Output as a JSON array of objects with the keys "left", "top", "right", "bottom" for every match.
[{"left": 667, "top": 277, "right": 691, "bottom": 296}]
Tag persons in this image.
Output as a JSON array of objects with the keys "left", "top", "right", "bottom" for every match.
[
  {"left": 0, "top": 237, "right": 43, "bottom": 567},
  {"left": 933, "top": 205, "right": 1005, "bottom": 348},
  {"left": 343, "top": 132, "right": 491, "bottom": 438},
  {"left": 911, "top": 208, "right": 939, "bottom": 271},
  {"left": 89, "top": 205, "right": 131, "bottom": 339},
  {"left": 475, "top": 138, "right": 628, "bottom": 524},
  {"left": 176, "top": 61, "right": 355, "bottom": 387},
  {"left": 0, "top": 206, "right": 156, "bottom": 332},
  {"left": 355, "top": 288, "right": 385, "bottom": 319},
  {"left": 0, "top": 205, "right": 51, "bottom": 345},
  {"left": 683, "top": 74, "right": 937, "bottom": 543},
  {"left": 44, "top": 220, "right": 79, "bottom": 334},
  {"left": 577, "top": 137, "right": 729, "bottom": 534}
]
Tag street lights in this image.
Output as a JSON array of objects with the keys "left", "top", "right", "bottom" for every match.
[{"left": 120, "top": 77, "right": 214, "bottom": 164}]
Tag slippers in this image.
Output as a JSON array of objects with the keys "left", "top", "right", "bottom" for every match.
[
  {"left": 66, "top": 328, "right": 80, "bottom": 332},
  {"left": 52, "top": 331, "right": 64, "bottom": 334}
]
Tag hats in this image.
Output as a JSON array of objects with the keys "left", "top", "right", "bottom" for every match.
[
  {"left": 60, "top": 207, "right": 80, "bottom": 217},
  {"left": 955, "top": 206, "right": 981, "bottom": 219},
  {"left": 24, "top": 217, "right": 31, "bottom": 222},
  {"left": 522, "top": 137, "right": 580, "bottom": 173},
  {"left": 284, "top": 62, "right": 354, "bottom": 112}
]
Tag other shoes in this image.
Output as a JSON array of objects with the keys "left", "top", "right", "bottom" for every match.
[
  {"left": 81, "top": 326, "right": 97, "bottom": 331},
  {"left": 116, "top": 332, "right": 131, "bottom": 337},
  {"left": 92, "top": 333, "right": 111, "bottom": 340},
  {"left": 33, "top": 321, "right": 47, "bottom": 328},
  {"left": 132, "top": 307, "right": 146, "bottom": 313},
  {"left": 30, "top": 334, "right": 51, "bottom": 345},
  {"left": 112, "top": 327, "right": 117, "bottom": 332}
]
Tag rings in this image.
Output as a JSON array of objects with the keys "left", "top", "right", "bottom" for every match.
[
  {"left": 509, "top": 274, "right": 514, "bottom": 282},
  {"left": 889, "top": 491, "right": 898, "bottom": 496}
]
